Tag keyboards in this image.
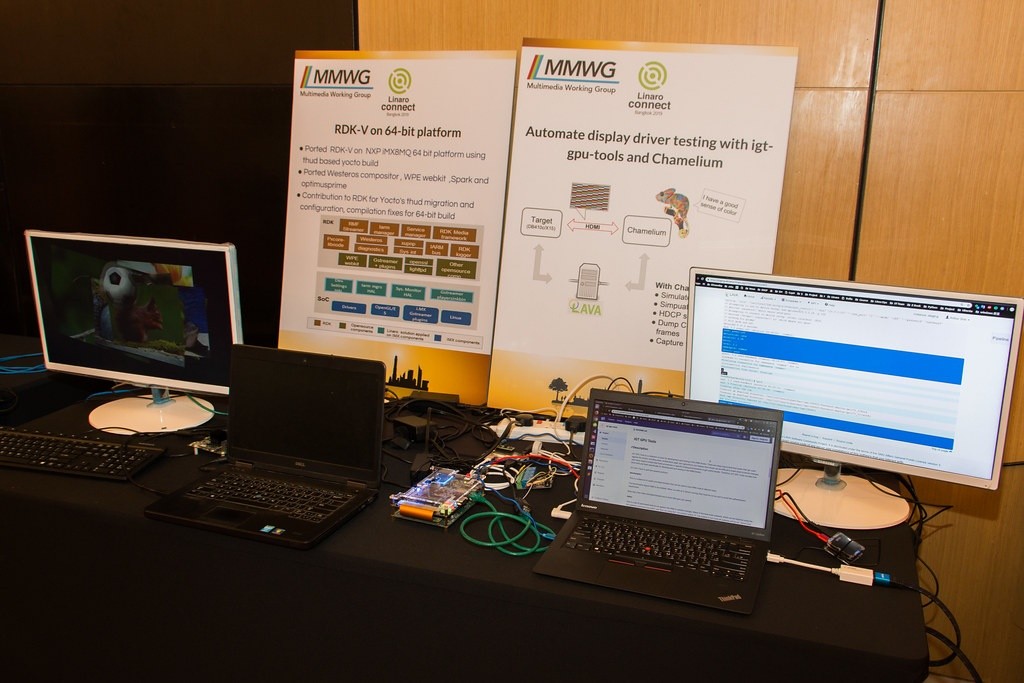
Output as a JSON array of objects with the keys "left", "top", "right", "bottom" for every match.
[{"left": 0, "top": 422, "right": 167, "bottom": 480}]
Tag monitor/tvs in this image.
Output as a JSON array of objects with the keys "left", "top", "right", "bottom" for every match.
[
  {"left": 23, "top": 228, "right": 244, "bottom": 396},
  {"left": 688, "top": 267, "right": 1024, "bottom": 491}
]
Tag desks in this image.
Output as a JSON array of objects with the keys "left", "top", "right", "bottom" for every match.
[{"left": 0, "top": 388, "right": 930, "bottom": 683}]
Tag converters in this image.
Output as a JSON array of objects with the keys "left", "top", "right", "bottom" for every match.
[
  {"left": 409, "top": 452, "right": 434, "bottom": 481},
  {"left": 392, "top": 413, "right": 439, "bottom": 439},
  {"left": 407, "top": 390, "right": 461, "bottom": 413},
  {"left": 831, "top": 564, "right": 874, "bottom": 586},
  {"left": 826, "top": 530, "right": 869, "bottom": 563}
]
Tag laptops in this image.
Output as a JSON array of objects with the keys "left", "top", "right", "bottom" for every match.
[
  {"left": 531, "top": 389, "right": 785, "bottom": 618},
  {"left": 147, "top": 345, "right": 386, "bottom": 550}
]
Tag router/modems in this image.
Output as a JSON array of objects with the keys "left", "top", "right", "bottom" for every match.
[{"left": 484, "top": 447, "right": 520, "bottom": 461}]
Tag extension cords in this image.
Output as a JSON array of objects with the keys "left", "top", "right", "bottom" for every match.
[{"left": 497, "top": 417, "right": 585, "bottom": 446}]
